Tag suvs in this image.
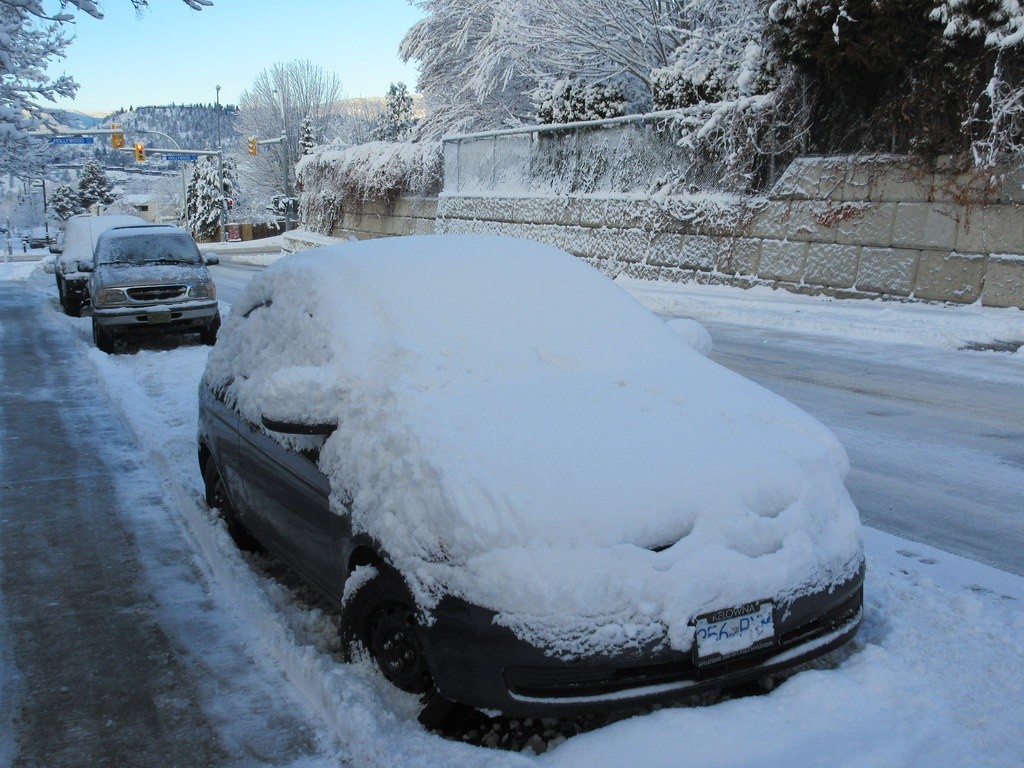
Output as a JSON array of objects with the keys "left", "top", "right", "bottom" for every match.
[
  {"left": 48, "top": 215, "right": 149, "bottom": 319},
  {"left": 88, "top": 223, "right": 222, "bottom": 354}
]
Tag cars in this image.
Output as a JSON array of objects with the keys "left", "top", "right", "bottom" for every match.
[
  {"left": 30, "top": 227, "right": 59, "bottom": 248},
  {"left": 193, "top": 232, "right": 865, "bottom": 728}
]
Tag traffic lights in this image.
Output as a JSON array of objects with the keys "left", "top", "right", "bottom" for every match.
[
  {"left": 214, "top": 197, "right": 232, "bottom": 210},
  {"left": 135, "top": 142, "right": 146, "bottom": 163},
  {"left": 248, "top": 135, "right": 259, "bottom": 156}
]
